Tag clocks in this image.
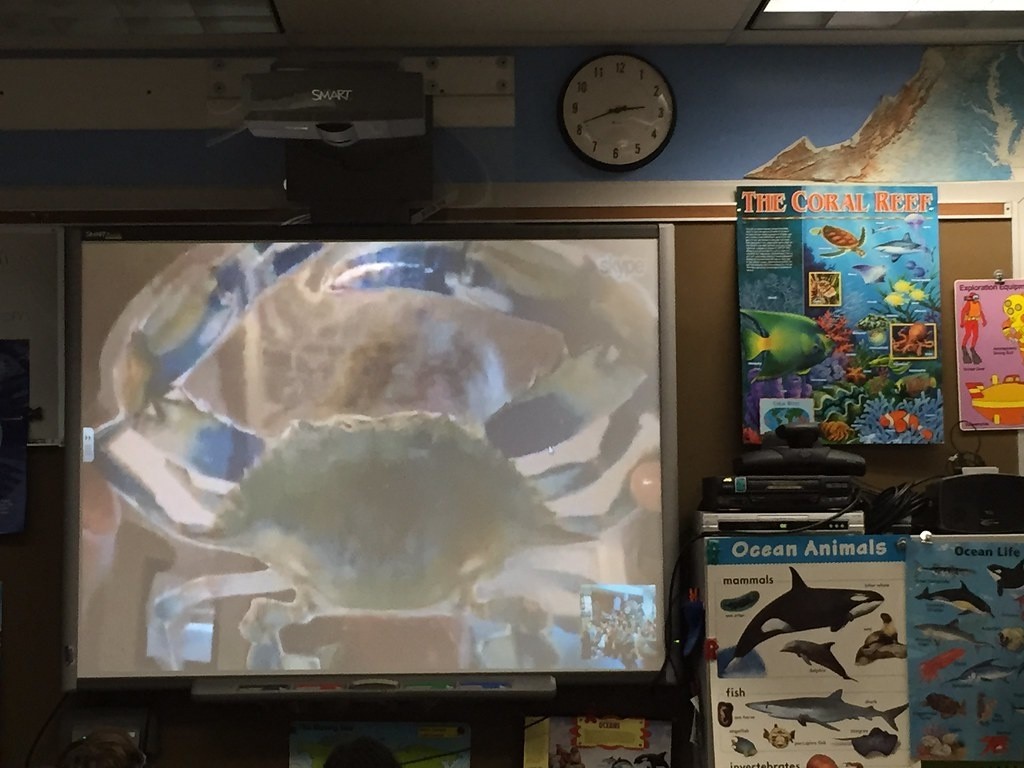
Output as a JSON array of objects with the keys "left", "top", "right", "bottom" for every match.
[{"left": 556, "top": 51, "right": 678, "bottom": 173}]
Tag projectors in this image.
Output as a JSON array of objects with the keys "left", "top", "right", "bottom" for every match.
[{"left": 244, "top": 70, "right": 426, "bottom": 146}]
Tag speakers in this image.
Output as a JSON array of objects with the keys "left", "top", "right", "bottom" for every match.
[{"left": 925, "top": 473, "right": 1024, "bottom": 534}]
